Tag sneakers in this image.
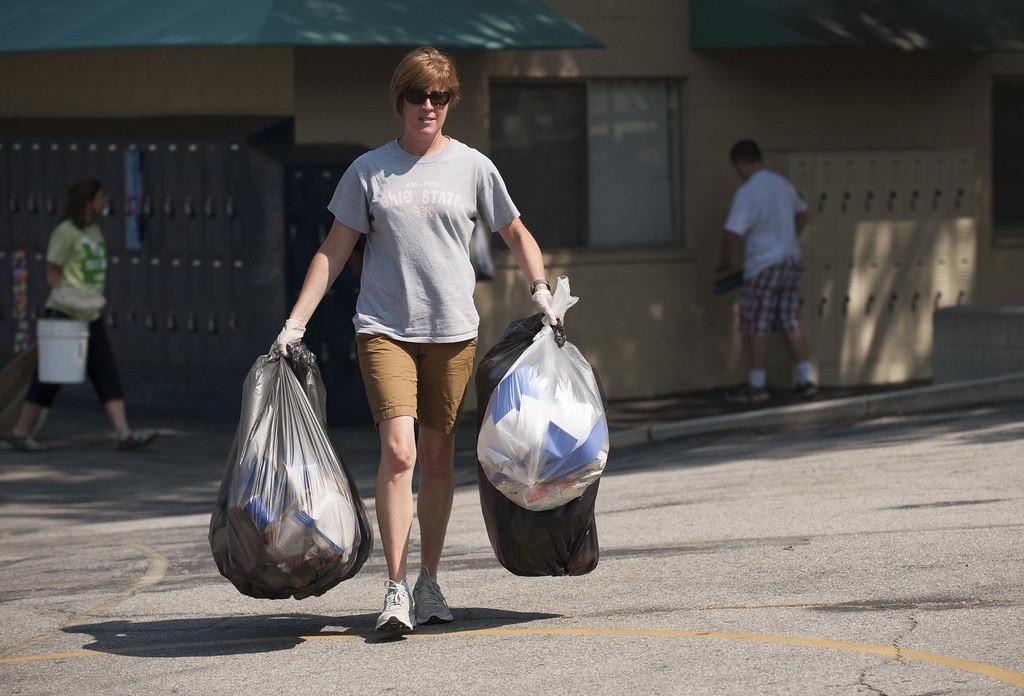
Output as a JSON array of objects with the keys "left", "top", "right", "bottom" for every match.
[
  {"left": 413, "top": 560, "right": 452, "bottom": 625},
  {"left": 375, "top": 579, "right": 418, "bottom": 633}
]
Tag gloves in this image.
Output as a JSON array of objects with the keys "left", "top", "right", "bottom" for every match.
[
  {"left": 530, "top": 291, "right": 560, "bottom": 343},
  {"left": 268, "top": 319, "right": 307, "bottom": 359}
]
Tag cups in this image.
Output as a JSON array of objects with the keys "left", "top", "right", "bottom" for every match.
[{"left": 243, "top": 495, "right": 274, "bottom": 528}]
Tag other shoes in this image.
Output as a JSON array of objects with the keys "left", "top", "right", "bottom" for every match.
[
  {"left": 5, "top": 428, "right": 49, "bottom": 451},
  {"left": 800, "top": 381, "right": 817, "bottom": 400},
  {"left": 117, "top": 431, "right": 157, "bottom": 451},
  {"left": 725, "top": 382, "right": 769, "bottom": 406}
]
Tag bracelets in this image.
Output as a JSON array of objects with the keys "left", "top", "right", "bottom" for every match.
[{"left": 530, "top": 279, "right": 551, "bottom": 295}]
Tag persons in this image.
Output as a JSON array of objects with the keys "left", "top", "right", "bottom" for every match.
[
  {"left": 715, "top": 138, "right": 818, "bottom": 402},
  {"left": 4, "top": 175, "right": 159, "bottom": 451},
  {"left": 272, "top": 47, "right": 557, "bottom": 632}
]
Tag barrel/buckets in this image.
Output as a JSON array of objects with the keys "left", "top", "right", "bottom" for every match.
[{"left": 36, "top": 318, "right": 89, "bottom": 384}]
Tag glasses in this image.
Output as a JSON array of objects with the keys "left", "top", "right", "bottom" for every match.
[{"left": 400, "top": 85, "right": 453, "bottom": 106}]
